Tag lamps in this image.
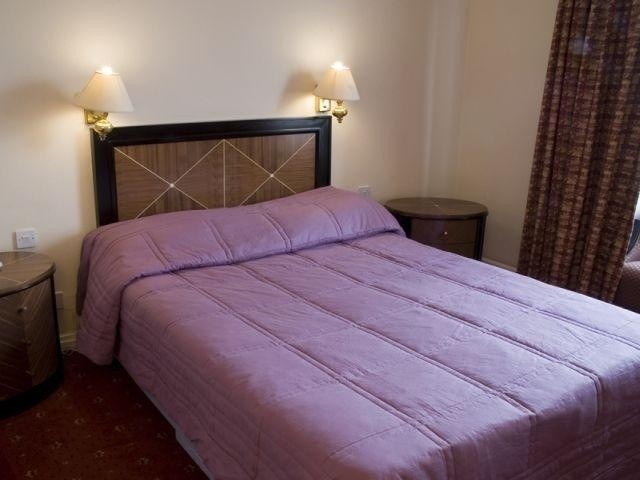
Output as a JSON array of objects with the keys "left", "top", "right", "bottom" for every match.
[
  {"left": 71, "top": 66, "right": 133, "bottom": 142},
  {"left": 310, "top": 62, "right": 360, "bottom": 124}
]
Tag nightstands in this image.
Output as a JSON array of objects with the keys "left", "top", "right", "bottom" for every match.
[
  {"left": 384, "top": 197, "right": 488, "bottom": 260},
  {"left": 0, "top": 252, "right": 63, "bottom": 420}
]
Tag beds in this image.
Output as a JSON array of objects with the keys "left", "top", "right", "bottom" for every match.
[{"left": 74, "top": 115, "right": 640, "bottom": 480}]
